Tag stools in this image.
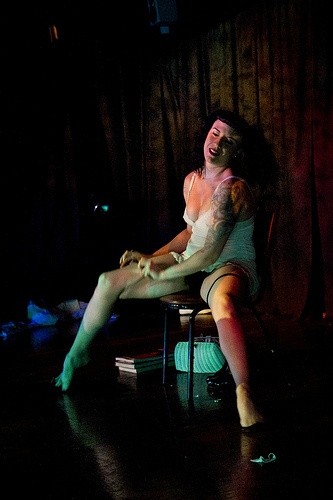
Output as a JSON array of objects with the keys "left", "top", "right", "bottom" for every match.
[{"left": 160, "top": 292, "right": 242, "bottom": 398}]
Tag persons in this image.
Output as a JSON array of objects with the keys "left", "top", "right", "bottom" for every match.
[{"left": 53, "top": 108, "right": 281, "bottom": 431}]
